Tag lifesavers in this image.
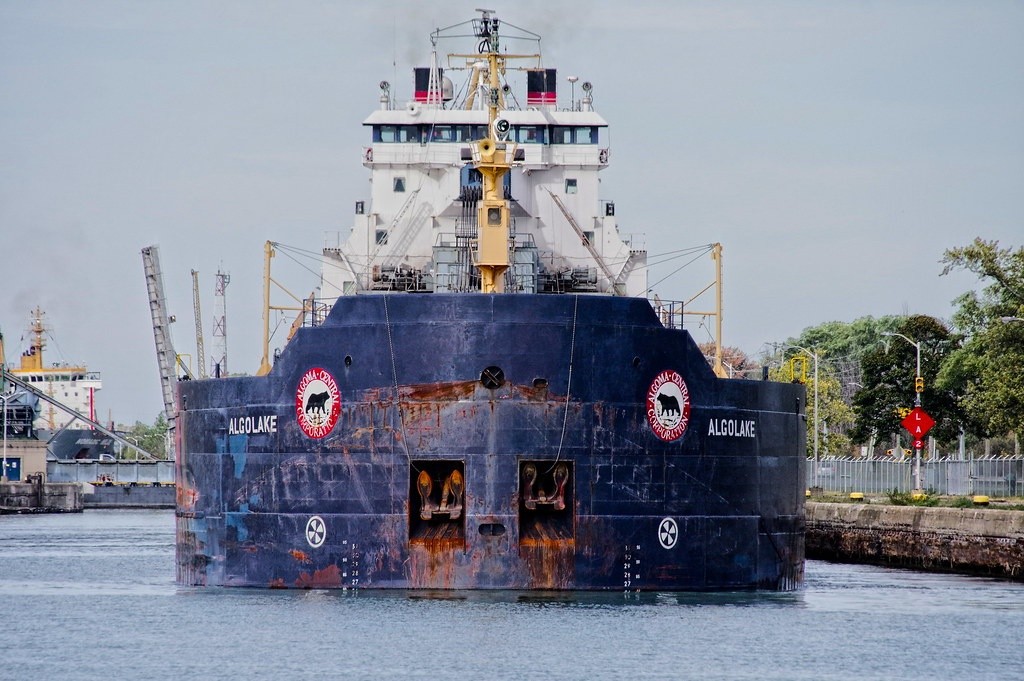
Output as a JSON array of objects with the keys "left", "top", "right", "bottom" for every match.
[
  {"left": 599, "top": 150, "right": 608, "bottom": 163},
  {"left": 366, "top": 148, "right": 373, "bottom": 161}
]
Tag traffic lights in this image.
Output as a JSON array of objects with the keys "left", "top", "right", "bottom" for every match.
[
  {"left": 887, "top": 449, "right": 894, "bottom": 456},
  {"left": 903, "top": 449, "right": 912, "bottom": 456},
  {"left": 916, "top": 377, "right": 924, "bottom": 393}
]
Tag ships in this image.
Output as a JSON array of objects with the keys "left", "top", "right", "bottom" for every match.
[
  {"left": 140, "top": 7, "right": 811, "bottom": 604},
  {"left": 0, "top": 305, "right": 178, "bottom": 514}
]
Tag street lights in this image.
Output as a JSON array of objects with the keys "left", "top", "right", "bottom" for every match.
[
  {"left": 784, "top": 344, "right": 819, "bottom": 489},
  {"left": 879, "top": 332, "right": 924, "bottom": 491}
]
